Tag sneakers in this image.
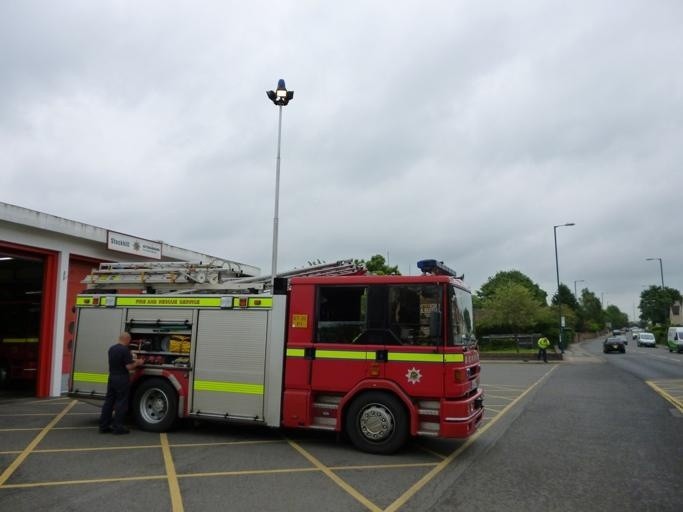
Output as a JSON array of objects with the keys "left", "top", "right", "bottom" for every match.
[
  {"left": 112, "top": 428, "right": 130, "bottom": 434},
  {"left": 100, "top": 427, "right": 113, "bottom": 433}
]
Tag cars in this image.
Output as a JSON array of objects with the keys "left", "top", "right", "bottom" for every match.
[
  {"left": 611, "top": 324, "right": 644, "bottom": 340},
  {"left": 637, "top": 332, "right": 655, "bottom": 347},
  {"left": 603, "top": 337, "right": 626, "bottom": 354}
]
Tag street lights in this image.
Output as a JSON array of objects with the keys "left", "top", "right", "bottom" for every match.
[
  {"left": 573, "top": 280, "right": 584, "bottom": 299},
  {"left": 645, "top": 257, "right": 665, "bottom": 296},
  {"left": 552, "top": 222, "right": 576, "bottom": 356},
  {"left": 264, "top": 77, "right": 293, "bottom": 295}
]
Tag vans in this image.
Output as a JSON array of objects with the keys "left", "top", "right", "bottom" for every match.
[{"left": 667, "top": 326, "right": 682, "bottom": 353}]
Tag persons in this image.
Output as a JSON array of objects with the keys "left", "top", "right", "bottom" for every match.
[
  {"left": 536, "top": 335, "right": 551, "bottom": 363},
  {"left": 94, "top": 331, "right": 146, "bottom": 437}
]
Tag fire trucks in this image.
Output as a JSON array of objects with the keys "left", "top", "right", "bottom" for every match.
[
  {"left": 66, "top": 260, "right": 484, "bottom": 453},
  {"left": 0, "top": 292, "right": 41, "bottom": 388}
]
